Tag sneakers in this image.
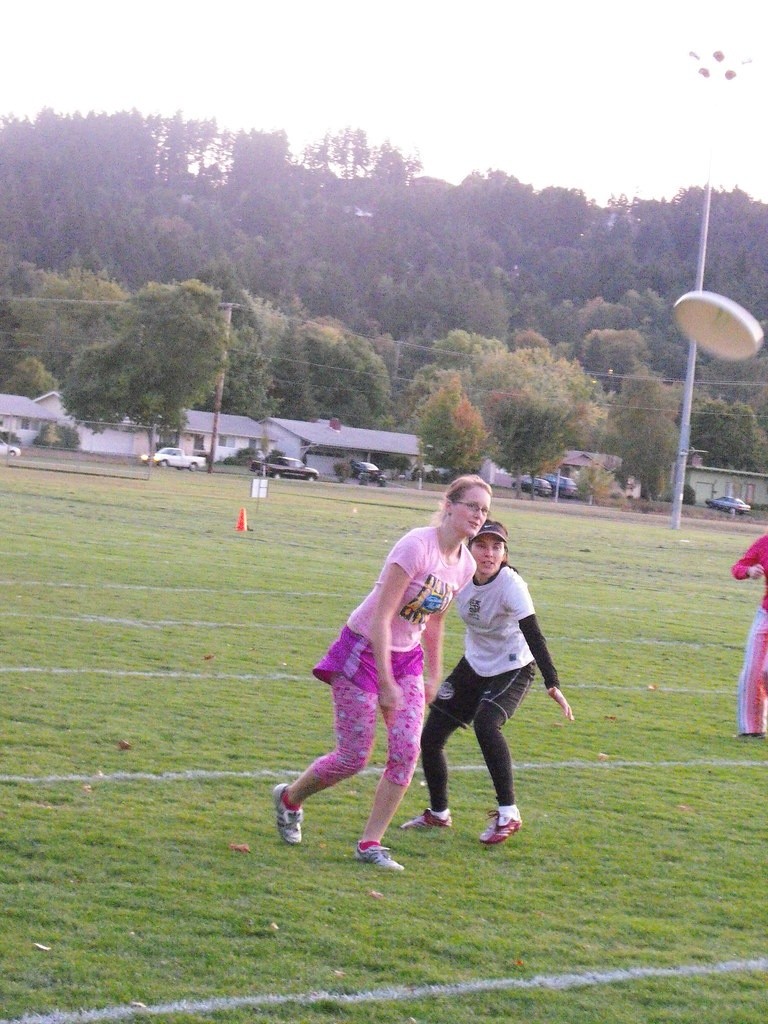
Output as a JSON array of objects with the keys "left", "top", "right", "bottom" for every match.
[
  {"left": 479, "top": 809, "right": 522, "bottom": 843},
  {"left": 400, "top": 808, "right": 452, "bottom": 829},
  {"left": 273, "top": 784, "right": 304, "bottom": 844},
  {"left": 355, "top": 840, "right": 404, "bottom": 872}
]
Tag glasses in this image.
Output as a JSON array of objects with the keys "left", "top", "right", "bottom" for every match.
[{"left": 454, "top": 500, "right": 490, "bottom": 515}]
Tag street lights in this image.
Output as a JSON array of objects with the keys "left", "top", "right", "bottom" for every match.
[{"left": 668, "top": 50, "right": 736, "bottom": 532}]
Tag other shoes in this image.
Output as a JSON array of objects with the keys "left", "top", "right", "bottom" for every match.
[{"left": 739, "top": 732, "right": 766, "bottom": 738}]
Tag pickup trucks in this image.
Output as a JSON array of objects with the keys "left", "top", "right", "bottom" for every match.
[
  {"left": 250, "top": 455, "right": 320, "bottom": 482},
  {"left": 140, "top": 447, "right": 207, "bottom": 472}
]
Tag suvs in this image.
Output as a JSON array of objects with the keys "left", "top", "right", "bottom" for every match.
[{"left": 541, "top": 473, "right": 578, "bottom": 499}]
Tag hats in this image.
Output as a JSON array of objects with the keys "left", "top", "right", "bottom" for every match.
[{"left": 470, "top": 523, "right": 507, "bottom": 544}]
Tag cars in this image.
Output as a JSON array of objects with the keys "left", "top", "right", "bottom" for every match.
[
  {"left": 512, "top": 476, "right": 553, "bottom": 497},
  {"left": 0, "top": 438, "right": 22, "bottom": 459},
  {"left": 352, "top": 462, "right": 386, "bottom": 482},
  {"left": 705, "top": 496, "right": 751, "bottom": 515}
]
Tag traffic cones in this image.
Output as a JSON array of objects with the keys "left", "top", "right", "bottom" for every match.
[{"left": 235, "top": 506, "right": 248, "bottom": 532}]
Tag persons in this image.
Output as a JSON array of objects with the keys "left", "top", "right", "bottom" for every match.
[
  {"left": 400, "top": 517, "right": 574, "bottom": 844},
  {"left": 271, "top": 471, "right": 492, "bottom": 873},
  {"left": 730, "top": 530, "right": 768, "bottom": 739}
]
